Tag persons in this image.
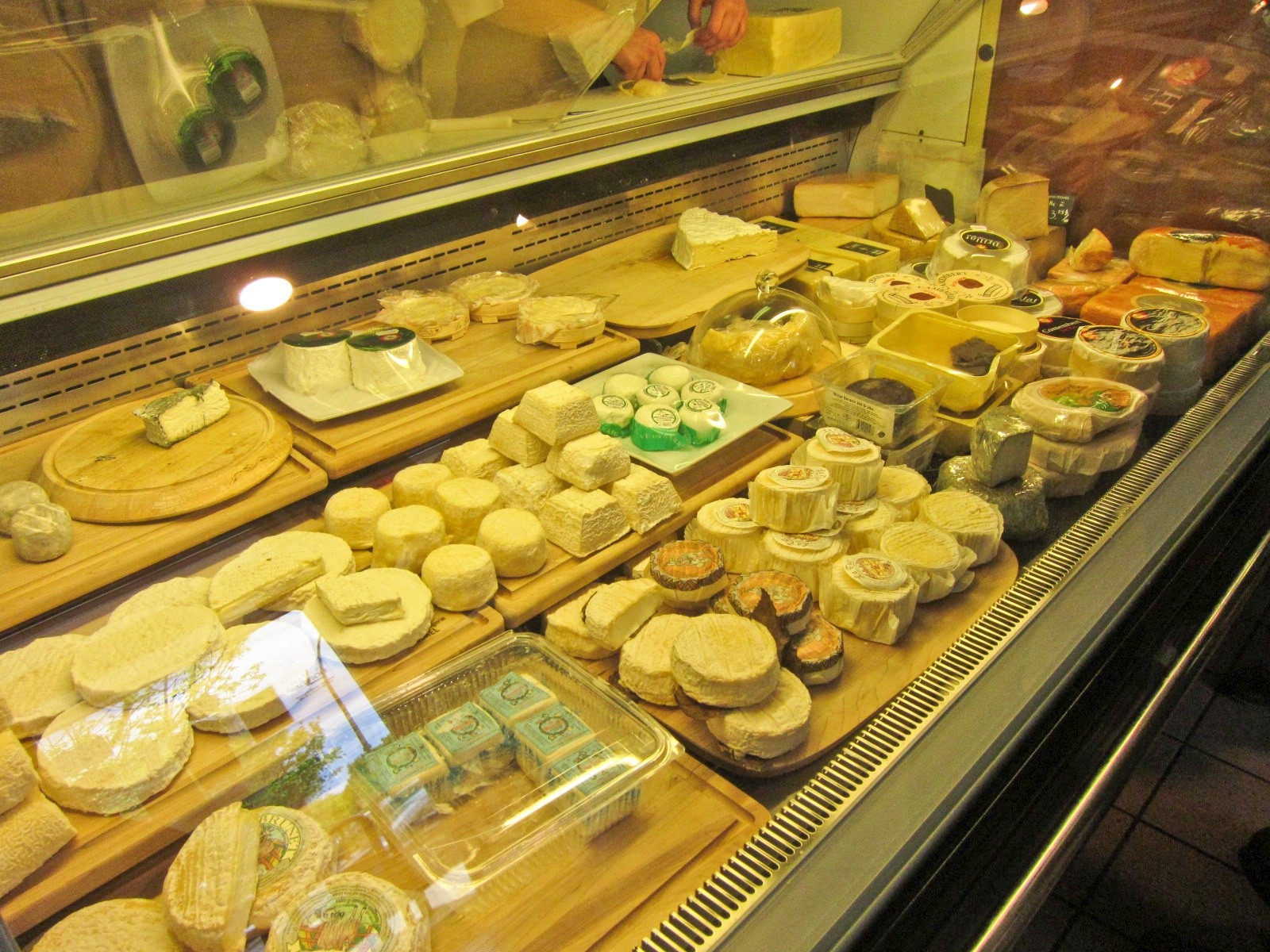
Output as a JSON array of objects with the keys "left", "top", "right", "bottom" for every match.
[{"left": 453, "top": 1, "right": 750, "bottom": 119}]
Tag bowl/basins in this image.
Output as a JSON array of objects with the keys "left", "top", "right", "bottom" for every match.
[
  {"left": 338, "top": 629, "right": 696, "bottom": 926},
  {"left": 813, "top": 304, "right": 1041, "bottom": 471}
]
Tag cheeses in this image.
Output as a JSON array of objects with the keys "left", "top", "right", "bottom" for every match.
[
  {"left": 0, "top": 172, "right": 1269, "bottom": 952},
  {"left": 711, "top": 4, "right": 843, "bottom": 79}
]
